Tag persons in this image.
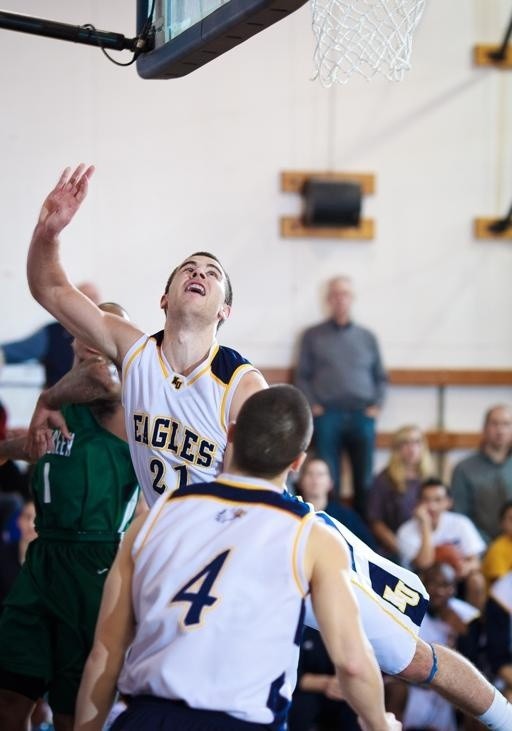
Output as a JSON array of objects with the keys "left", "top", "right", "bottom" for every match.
[
  {"left": 289, "top": 272, "right": 390, "bottom": 515},
  {"left": 2, "top": 502, "right": 39, "bottom": 580},
  {"left": 296, "top": 456, "right": 380, "bottom": 553},
  {"left": 0, "top": 280, "right": 107, "bottom": 391},
  {"left": 0, "top": 302, "right": 144, "bottom": 731},
  {"left": 482, "top": 501, "right": 511, "bottom": 580},
  {"left": 69, "top": 379, "right": 406, "bottom": 730},
  {"left": 398, "top": 478, "right": 487, "bottom": 608},
  {"left": 484, "top": 574, "right": 512, "bottom": 684},
  {"left": 25, "top": 161, "right": 512, "bottom": 731},
  {"left": 366, "top": 425, "right": 431, "bottom": 553},
  {"left": 451, "top": 405, "right": 512, "bottom": 540},
  {"left": 389, "top": 562, "right": 480, "bottom": 731}
]
